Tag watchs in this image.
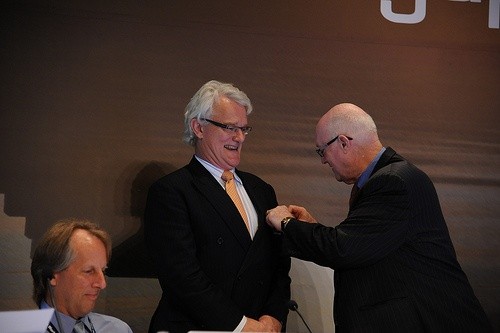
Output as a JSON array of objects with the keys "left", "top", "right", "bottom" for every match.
[{"left": 281, "top": 217, "right": 293, "bottom": 231}]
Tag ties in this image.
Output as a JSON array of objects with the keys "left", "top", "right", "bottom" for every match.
[
  {"left": 222, "top": 169, "right": 250, "bottom": 233},
  {"left": 73, "top": 322, "right": 86, "bottom": 333}
]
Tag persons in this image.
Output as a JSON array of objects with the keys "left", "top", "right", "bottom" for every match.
[
  {"left": 148, "top": 81, "right": 292, "bottom": 333},
  {"left": 31, "top": 219, "right": 132, "bottom": 333},
  {"left": 266, "top": 104, "right": 492, "bottom": 333}
]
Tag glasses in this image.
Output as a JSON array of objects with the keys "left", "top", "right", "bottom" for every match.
[
  {"left": 315, "top": 135, "right": 353, "bottom": 158},
  {"left": 196, "top": 117, "right": 252, "bottom": 135}
]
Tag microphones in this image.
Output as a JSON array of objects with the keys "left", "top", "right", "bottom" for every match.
[{"left": 287, "top": 300, "right": 312, "bottom": 333}]
